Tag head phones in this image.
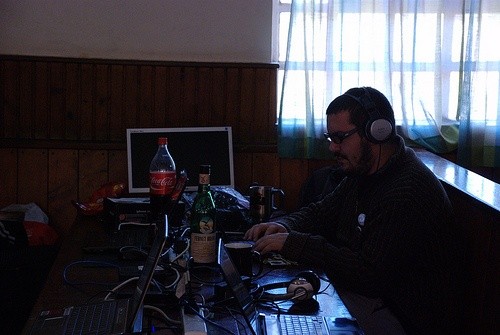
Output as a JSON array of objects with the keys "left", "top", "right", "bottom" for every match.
[
  {"left": 345, "top": 87, "right": 394, "bottom": 144},
  {"left": 257, "top": 270, "right": 320, "bottom": 305}
]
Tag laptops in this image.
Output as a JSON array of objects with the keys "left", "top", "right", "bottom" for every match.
[
  {"left": 29, "top": 215, "right": 168, "bottom": 335},
  {"left": 217, "top": 239, "right": 364, "bottom": 335}
]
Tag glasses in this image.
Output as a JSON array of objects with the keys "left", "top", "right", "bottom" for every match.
[{"left": 323, "top": 128, "right": 362, "bottom": 144}]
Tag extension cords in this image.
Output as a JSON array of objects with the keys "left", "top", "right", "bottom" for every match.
[{"left": 182, "top": 303, "right": 207, "bottom": 335}]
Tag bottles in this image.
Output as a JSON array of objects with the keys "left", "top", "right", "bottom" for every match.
[
  {"left": 189, "top": 165, "right": 217, "bottom": 279},
  {"left": 150, "top": 138, "right": 176, "bottom": 224}
]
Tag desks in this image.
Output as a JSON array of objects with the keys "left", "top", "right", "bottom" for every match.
[{"left": 21, "top": 198, "right": 362, "bottom": 335}]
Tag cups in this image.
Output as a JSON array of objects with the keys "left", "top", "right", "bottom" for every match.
[
  {"left": 250, "top": 185, "right": 285, "bottom": 219},
  {"left": 224, "top": 242, "right": 262, "bottom": 278}
]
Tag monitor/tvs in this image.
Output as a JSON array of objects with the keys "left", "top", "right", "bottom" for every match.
[{"left": 126, "top": 127, "right": 234, "bottom": 194}]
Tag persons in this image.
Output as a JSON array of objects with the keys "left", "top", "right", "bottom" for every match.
[{"left": 243, "top": 87, "right": 467, "bottom": 335}]
